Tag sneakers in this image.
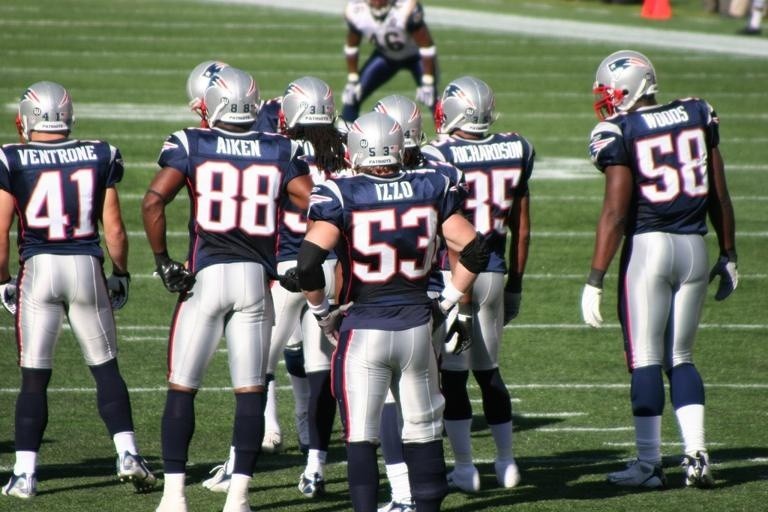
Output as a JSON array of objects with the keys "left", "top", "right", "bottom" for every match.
[
  {"left": 115, "top": 449, "right": 161, "bottom": 494},
  {"left": 737, "top": 25, "right": 761, "bottom": 36},
  {"left": 681, "top": 448, "right": 711, "bottom": 487},
  {"left": 158, "top": 455, "right": 518, "bottom": 512},
  {"left": 607, "top": 460, "right": 666, "bottom": 489},
  {"left": 1, "top": 472, "right": 38, "bottom": 497}
]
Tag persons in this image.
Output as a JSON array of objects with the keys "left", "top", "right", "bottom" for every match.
[
  {"left": 580, "top": 49, "right": 739, "bottom": 490},
  {"left": 0, "top": 80, "right": 156, "bottom": 501},
  {"left": 338, "top": 0, "right": 441, "bottom": 126},
  {"left": 140, "top": 61, "right": 536, "bottom": 512}
]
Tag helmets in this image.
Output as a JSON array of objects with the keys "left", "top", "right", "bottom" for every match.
[
  {"left": 367, "top": 1, "right": 394, "bottom": 18},
  {"left": 280, "top": 74, "right": 333, "bottom": 128},
  {"left": 593, "top": 50, "right": 657, "bottom": 122},
  {"left": 346, "top": 111, "right": 405, "bottom": 169},
  {"left": 372, "top": 95, "right": 423, "bottom": 149},
  {"left": 15, "top": 81, "right": 75, "bottom": 141},
  {"left": 183, "top": 58, "right": 262, "bottom": 131},
  {"left": 439, "top": 75, "right": 496, "bottom": 133}
]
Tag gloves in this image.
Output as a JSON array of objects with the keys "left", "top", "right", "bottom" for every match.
[
  {"left": 504, "top": 274, "right": 521, "bottom": 325},
  {"left": 107, "top": 272, "right": 130, "bottom": 310},
  {"left": 581, "top": 268, "right": 604, "bottom": 329},
  {"left": 0, "top": 276, "right": 17, "bottom": 316},
  {"left": 158, "top": 260, "right": 196, "bottom": 292},
  {"left": 313, "top": 302, "right": 353, "bottom": 347},
  {"left": 430, "top": 295, "right": 454, "bottom": 336},
  {"left": 444, "top": 302, "right": 474, "bottom": 357},
  {"left": 708, "top": 250, "right": 739, "bottom": 301}
]
